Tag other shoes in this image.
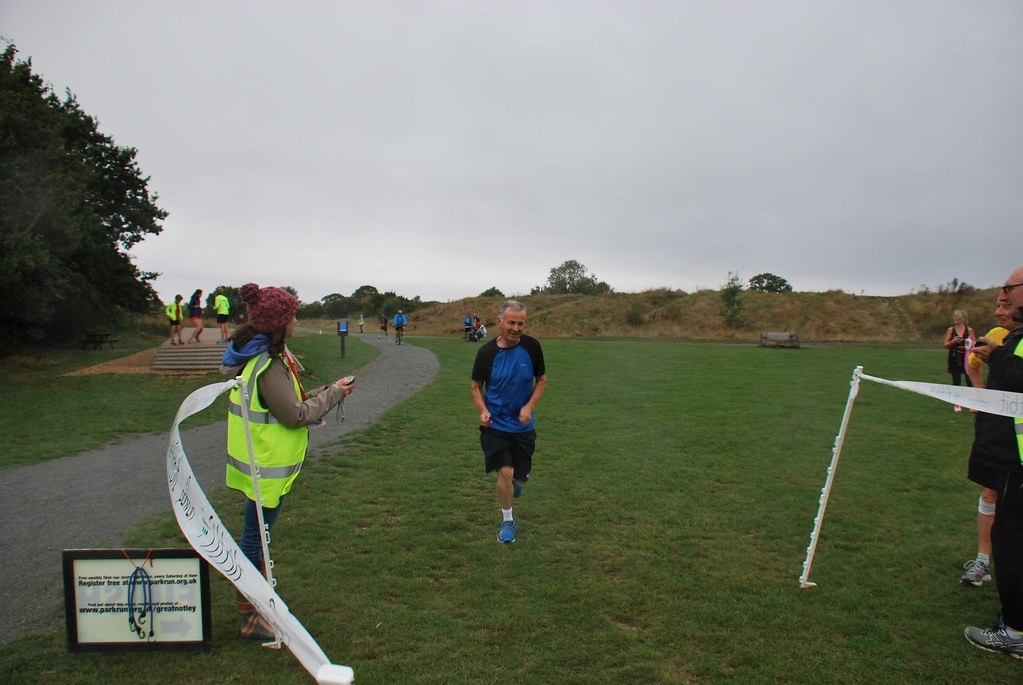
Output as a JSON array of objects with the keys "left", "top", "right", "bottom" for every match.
[
  {"left": 954, "top": 405, "right": 961, "bottom": 412},
  {"left": 970, "top": 408, "right": 977, "bottom": 413},
  {"left": 171, "top": 339, "right": 176, "bottom": 344},
  {"left": 179, "top": 339, "right": 185, "bottom": 344},
  {"left": 195, "top": 336, "right": 200, "bottom": 342},
  {"left": 188, "top": 340, "right": 195, "bottom": 343},
  {"left": 216, "top": 338, "right": 228, "bottom": 344}
]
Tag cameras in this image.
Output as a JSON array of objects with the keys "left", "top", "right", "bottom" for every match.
[{"left": 345, "top": 375, "right": 355, "bottom": 386}]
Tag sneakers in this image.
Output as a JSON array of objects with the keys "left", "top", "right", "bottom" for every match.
[
  {"left": 959, "top": 558, "right": 992, "bottom": 586},
  {"left": 964, "top": 615, "right": 1023, "bottom": 661},
  {"left": 498, "top": 521, "right": 517, "bottom": 543},
  {"left": 512, "top": 479, "right": 521, "bottom": 499}
]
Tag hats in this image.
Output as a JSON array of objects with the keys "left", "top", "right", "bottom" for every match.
[{"left": 238, "top": 283, "right": 299, "bottom": 343}]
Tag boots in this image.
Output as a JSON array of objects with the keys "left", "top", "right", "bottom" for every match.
[{"left": 236, "top": 599, "right": 274, "bottom": 639}]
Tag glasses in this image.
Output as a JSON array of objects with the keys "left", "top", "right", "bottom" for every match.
[{"left": 1002, "top": 281, "right": 1023, "bottom": 294}]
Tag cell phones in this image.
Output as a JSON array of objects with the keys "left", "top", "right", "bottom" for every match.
[{"left": 975, "top": 341, "right": 986, "bottom": 347}]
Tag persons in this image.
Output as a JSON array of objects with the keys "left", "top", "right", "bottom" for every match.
[
  {"left": 219, "top": 284, "right": 355, "bottom": 641},
  {"left": 379, "top": 313, "right": 388, "bottom": 337},
  {"left": 942, "top": 268, "right": 1023, "bottom": 663},
  {"left": 464, "top": 313, "right": 480, "bottom": 343},
  {"left": 393, "top": 310, "right": 407, "bottom": 341},
  {"left": 165, "top": 294, "right": 185, "bottom": 345},
  {"left": 358, "top": 315, "right": 364, "bottom": 333},
  {"left": 187, "top": 289, "right": 204, "bottom": 344},
  {"left": 470, "top": 300, "right": 547, "bottom": 543},
  {"left": 212, "top": 288, "right": 230, "bottom": 344}
]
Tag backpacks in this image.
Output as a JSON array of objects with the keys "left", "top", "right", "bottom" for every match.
[{"left": 465, "top": 316, "right": 471, "bottom": 325}]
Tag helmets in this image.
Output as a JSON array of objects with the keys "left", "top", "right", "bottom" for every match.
[{"left": 398, "top": 310, "right": 402, "bottom": 314}]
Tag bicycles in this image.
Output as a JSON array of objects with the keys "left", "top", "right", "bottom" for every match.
[
  {"left": 393, "top": 323, "right": 405, "bottom": 345},
  {"left": 464, "top": 326, "right": 479, "bottom": 343}
]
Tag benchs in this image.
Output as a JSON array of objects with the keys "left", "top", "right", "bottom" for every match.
[
  {"left": 83, "top": 333, "right": 117, "bottom": 349},
  {"left": 760, "top": 332, "right": 799, "bottom": 348},
  {"left": 79, "top": 339, "right": 119, "bottom": 349}
]
